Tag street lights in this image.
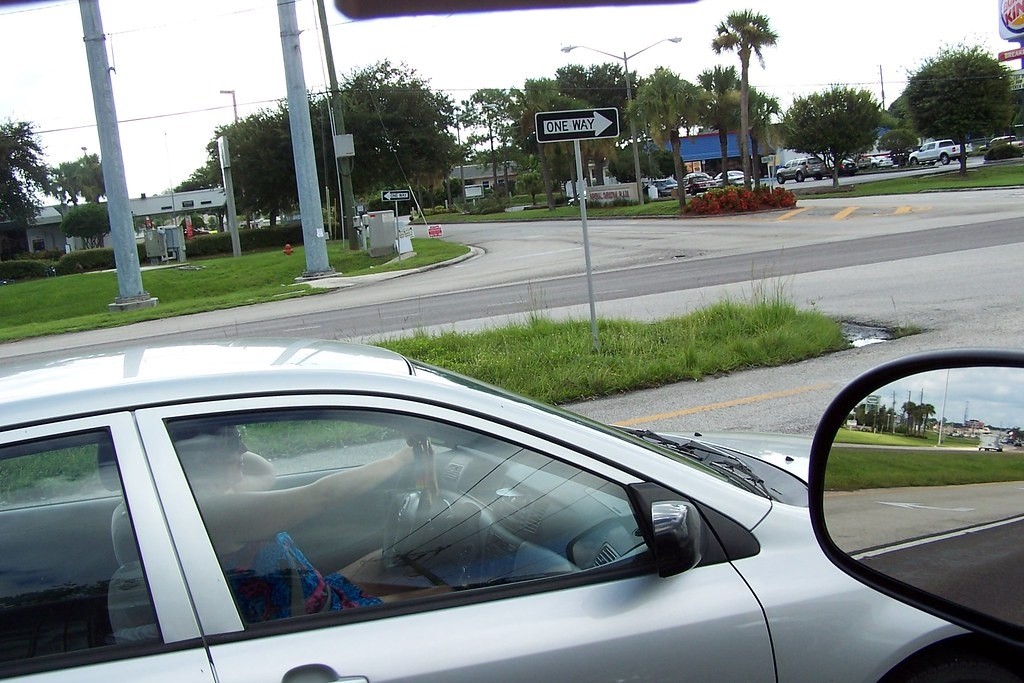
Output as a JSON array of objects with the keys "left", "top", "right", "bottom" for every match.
[{"left": 561, "top": 35, "right": 682, "bottom": 204}]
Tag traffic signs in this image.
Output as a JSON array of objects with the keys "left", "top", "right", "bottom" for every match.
[
  {"left": 380, "top": 190, "right": 411, "bottom": 203},
  {"left": 535, "top": 108, "right": 621, "bottom": 144}
]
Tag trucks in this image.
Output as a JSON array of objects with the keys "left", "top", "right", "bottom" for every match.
[{"left": 978, "top": 434, "right": 1003, "bottom": 453}]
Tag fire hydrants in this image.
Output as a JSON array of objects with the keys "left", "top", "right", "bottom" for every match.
[{"left": 284, "top": 243, "right": 295, "bottom": 255}]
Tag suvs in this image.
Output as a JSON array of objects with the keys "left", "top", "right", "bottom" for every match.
[{"left": 776, "top": 157, "right": 827, "bottom": 184}]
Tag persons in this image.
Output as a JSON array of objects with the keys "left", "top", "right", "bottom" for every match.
[{"left": 169, "top": 419, "right": 453, "bottom": 623}]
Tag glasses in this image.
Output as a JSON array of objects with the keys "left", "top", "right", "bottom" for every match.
[{"left": 224, "top": 426, "right": 247, "bottom": 451}]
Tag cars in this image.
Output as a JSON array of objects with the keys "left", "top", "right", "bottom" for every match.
[
  {"left": 682, "top": 172, "right": 718, "bottom": 195},
  {"left": 714, "top": 170, "right": 754, "bottom": 189},
  {"left": 857, "top": 155, "right": 895, "bottom": 170},
  {"left": 0, "top": 337, "right": 1024, "bottom": 683},
  {"left": 827, "top": 160, "right": 858, "bottom": 176},
  {"left": 642, "top": 179, "right": 687, "bottom": 198}
]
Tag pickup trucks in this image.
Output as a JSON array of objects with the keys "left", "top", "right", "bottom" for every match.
[
  {"left": 979, "top": 136, "right": 1024, "bottom": 155},
  {"left": 908, "top": 139, "right": 973, "bottom": 166}
]
